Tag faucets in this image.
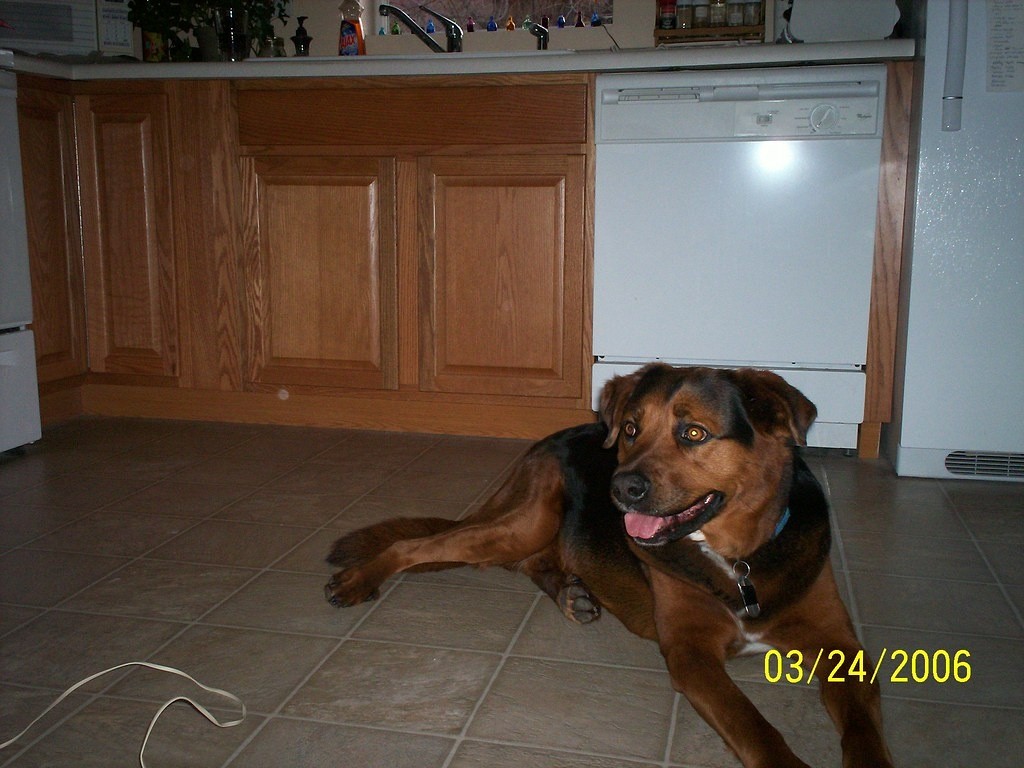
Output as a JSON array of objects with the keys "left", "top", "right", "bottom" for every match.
[{"left": 378, "top": 4, "right": 464, "bottom": 54}]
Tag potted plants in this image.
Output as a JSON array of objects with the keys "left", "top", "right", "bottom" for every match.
[{"left": 127, "top": 0, "right": 291, "bottom": 62}]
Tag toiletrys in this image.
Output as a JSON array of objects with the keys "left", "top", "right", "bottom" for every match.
[
  {"left": 290, "top": 15, "right": 314, "bottom": 57},
  {"left": 338, "top": 0, "right": 366, "bottom": 57}
]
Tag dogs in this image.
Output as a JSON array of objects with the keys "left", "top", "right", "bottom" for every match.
[{"left": 324, "top": 362, "right": 894, "bottom": 768}]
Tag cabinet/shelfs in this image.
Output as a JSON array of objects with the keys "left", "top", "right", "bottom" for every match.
[{"left": 17, "top": 67, "right": 598, "bottom": 440}]
